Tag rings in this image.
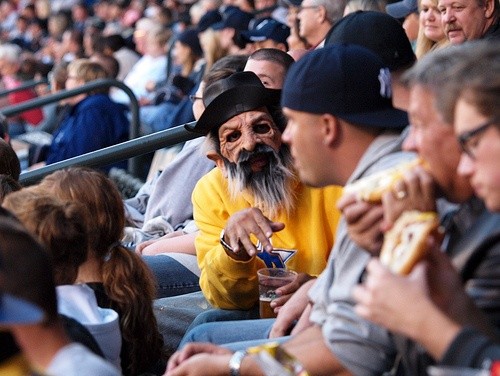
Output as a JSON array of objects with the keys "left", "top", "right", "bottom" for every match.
[{"left": 397, "top": 190, "right": 407, "bottom": 199}]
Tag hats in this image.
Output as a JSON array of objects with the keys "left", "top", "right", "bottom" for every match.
[
  {"left": 241, "top": 19, "right": 291, "bottom": 41},
  {"left": 386, "top": 0, "right": 418, "bottom": 18},
  {"left": 212, "top": 9, "right": 242, "bottom": 29},
  {"left": 284, "top": 47, "right": 407, "bottom": 130},
  {"left": 325, "top": 11, "right": 418, "bottom": 64},
  {"left": 179, "top": 30, "right": 203, "bottom": 54},
  {"left": 184, "top": 71, "right": 282, "bottom": 134},
  {"left": 193, "top": 12, "right": 218, "bottom": 31}
]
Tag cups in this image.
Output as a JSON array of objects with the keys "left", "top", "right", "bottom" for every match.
[{"left": 257, "top": 267, "right": 297, "bottom": 319}]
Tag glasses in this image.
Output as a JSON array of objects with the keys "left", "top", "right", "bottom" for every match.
[
  {"left": 300, "top": 5, "right": 319, "bottom": 12},
  {"left": 459, "top": 117, "right": 500, "bottom": 159}
]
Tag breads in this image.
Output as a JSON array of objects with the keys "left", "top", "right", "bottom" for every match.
[{"left": 343, "top": 158, "right": 425, "bottom": 202}]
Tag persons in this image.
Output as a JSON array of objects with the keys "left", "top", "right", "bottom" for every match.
[{"left": 0, "top": 0, "right": 499, "bottom": 376}]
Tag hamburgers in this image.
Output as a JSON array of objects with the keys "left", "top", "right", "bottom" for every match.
[{"left": 379, "top": 209, "right": 441, "bottom": 275}]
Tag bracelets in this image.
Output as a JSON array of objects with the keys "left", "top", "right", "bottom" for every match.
[{"left": 230, "top": 350, "right": 248, "bottom": 376}]
